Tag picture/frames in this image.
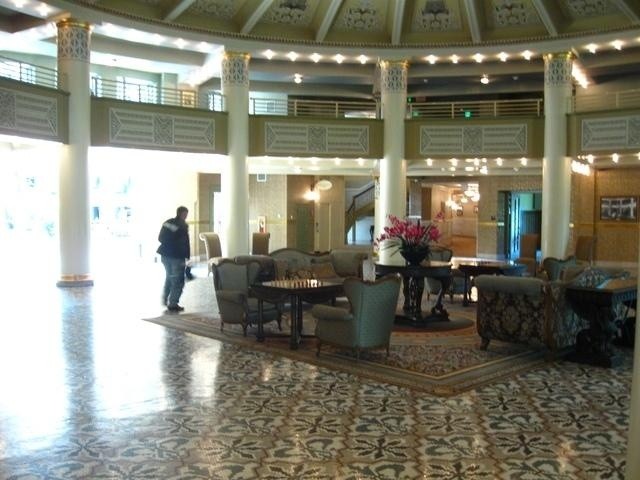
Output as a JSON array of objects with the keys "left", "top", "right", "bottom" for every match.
[{"left": 599, "top": 195, "right": 637, "bottom": 224}]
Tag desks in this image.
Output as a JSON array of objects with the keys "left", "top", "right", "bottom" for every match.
[
  {"left": 248, "top": 278, "right": 344, "bottom": 350},
  {"left": 374, "top": 259, "right": 454, "bottom": 329},
  {"left": 458, "top": 261, "right": 515, "bottom": 307},
  {"left": 563, "top": 275, "right": 638, "bottom": 369}
]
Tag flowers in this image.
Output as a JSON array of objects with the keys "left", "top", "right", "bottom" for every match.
[{"left": 376, "top": 210, "right": 447, "bottom": 258}]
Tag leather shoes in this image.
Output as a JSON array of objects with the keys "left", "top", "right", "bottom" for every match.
[{"left": 160, "top": 297, "right": 184, "bottom": 311}]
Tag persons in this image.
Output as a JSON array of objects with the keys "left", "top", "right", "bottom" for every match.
[{"left": 155, "top": 207, "right": 191, "bottom": 311}]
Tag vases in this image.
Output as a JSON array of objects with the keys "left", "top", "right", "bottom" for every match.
[{"left": 400, "top": 249, "right": 431, "bottom": 265}]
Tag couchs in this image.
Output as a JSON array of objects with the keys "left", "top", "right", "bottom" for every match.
[
  {"left": 233, "top": 247, "right": 379, "bottom": 314},
  {"left": 473, "top": 267, "right": 632, "bottom": 364}
]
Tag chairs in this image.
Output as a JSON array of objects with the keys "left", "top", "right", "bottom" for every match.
[
  {"left": 311, "top": 273, "right": 402, "bottom": 365},
  {"left": 513, "top": 233, "right": 597, "bottom": 281},
  {"left": 198, "top": 231, "right": 270, "bottom": 277},
  {"left": 211, "top": 259, "right": 285, "bottom": 337}
]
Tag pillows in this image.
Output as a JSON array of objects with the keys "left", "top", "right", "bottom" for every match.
[{"left": 286, "top": 262, "right": 338, "bottom": 281}]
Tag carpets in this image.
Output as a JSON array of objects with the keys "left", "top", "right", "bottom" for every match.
[{"left": 140, "top": 289, "right": 575, "bottom": 398}]
